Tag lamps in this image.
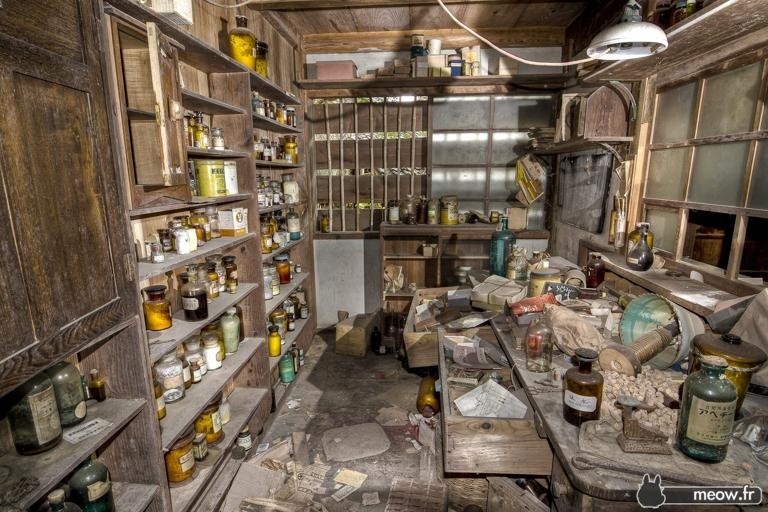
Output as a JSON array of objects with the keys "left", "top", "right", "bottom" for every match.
[{"left": 586, "top": 0, "right": 669, "bottom": 61}]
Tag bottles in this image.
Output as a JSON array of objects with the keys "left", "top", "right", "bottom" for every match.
[
  {"left": 252, "top": 88, "right": 309, "bottom": 385},
  {"left": 506, "top": 255, "right": 527, "bottom": 280},
  {"left": 586, "top": 253, "right": 605, "bottom": 289},
  {"left": 137, "top": 109, "right": 252, "bottom": 484},
  {"left": 488, "top": 215, "right": 517, "bottom": 275},
  {"left": 230, "top": 17, "right": 256, "bottom": 70},
  {"left": 526, "top": 250, "right": 543, "bottom": 282},
  {"left": 627, "top": 221, "right": 653, "bottom": 254},
  {"left": 388, "top": 192, "right": 438, "bottom": 226},
  {"left": 7, "top": 361, "right": 117, "bottom": 512},
  {"left": 655, "top": 0, "right": 697, "bottom": 32},
  {"left": 255, "top": 39, "right": 268, "bottom": 78},
  {"left": 464, "top": 60, "right": 480, "bottom": 76},
  {"left": 677, "top": 357, "right": 738, "bottom": 461},
  {"left": 320, "top": 215, "right": 331, "bottom": 233},
  {"left": 373, "top": 312, "right": 406, "bottom": 356},
  {"left": 417, "top": 376, "right": 440, "bottom": 417},
  {"left": 564, "top": 349, "right": 605, "bottom": 423},
  {"left": 626, "top": 224, "right": 654, "bottom": 271}
]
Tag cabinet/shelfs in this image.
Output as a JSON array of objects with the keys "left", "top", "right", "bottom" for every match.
[
  {"left": 1, "top": 0, "right": 173, "bottom": 512},
  {"left": 248, "top": 69, "right": 318, "bottom": 415},
  {"left": 380, "top": 224, "right": 499, "bottom": 354},
  {"left": 96, "top": 0, "right": 273, "bottom": 512}
]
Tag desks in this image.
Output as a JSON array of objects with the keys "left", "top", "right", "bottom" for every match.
[{"left": 402, "top": 273, "right": 767, "bottom": 512}]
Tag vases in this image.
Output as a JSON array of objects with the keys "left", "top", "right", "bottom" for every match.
[{"left": 624, "top": 222, "right": 655, "bottom": 272}]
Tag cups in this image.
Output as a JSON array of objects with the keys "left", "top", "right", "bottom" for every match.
[{"left": 522, "top": 315, "right": 554, "bottom": 375}]
